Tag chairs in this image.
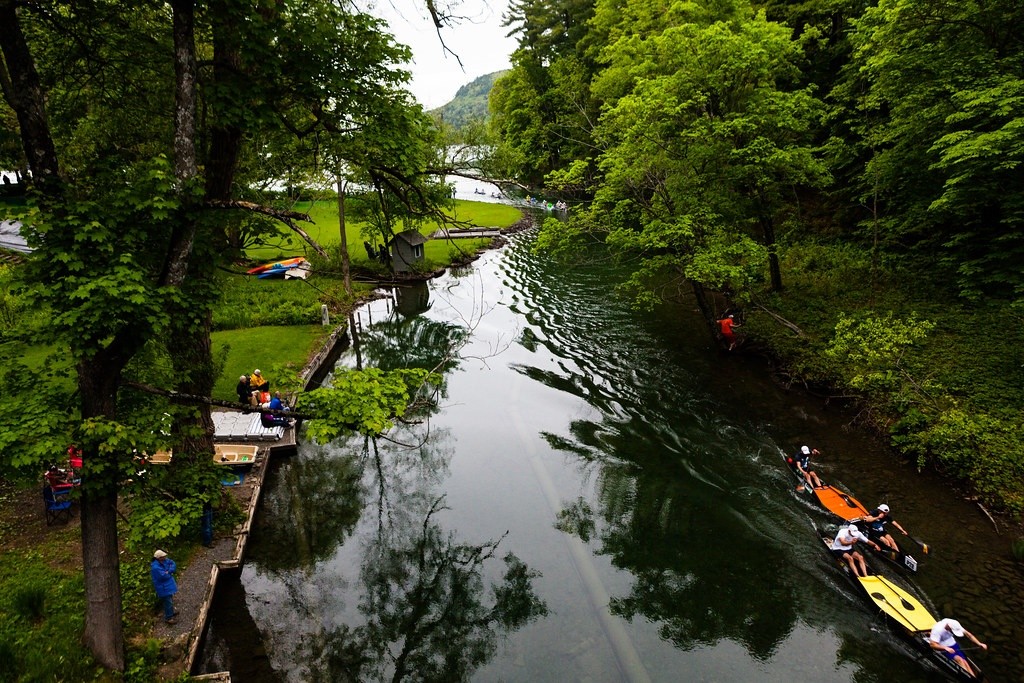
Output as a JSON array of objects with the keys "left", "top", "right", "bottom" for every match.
[
  {"left": 42, "top": 486, "right": 73, "bottom": 527},
  {"left": 44, "top": 469, "right": 80, "bottom": 499},
  {"left": 68, "top": 445, "right": 82, "bottom": 475}
]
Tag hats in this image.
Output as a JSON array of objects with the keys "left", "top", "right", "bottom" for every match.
[
  {"left": 254, "top": 369, "right": 260, "bottom": 373},
  {"left": 849, "top": 524, "right": 859, "bottom": 536},
  {"left": 154, "top": 550, "right": 167, "bottom": 558},
  {"left": 878, "top": 504, "right": 890, "bottom": 512},
  {"left": 240, "top": 376, "right": 246, "bottom": 381},
  {"left": 801, "top": 446, "right": 810, "bottom": 454},
  {"left": 48, "top": 465, "right": 57, "bottom": 472},
  {"left": 947, "top": 620, "right": 964, "bottom": 637},
  {"left": 262, "top": 403, "right": 269, "bottom": 408},
  {"left": 728, "top": 315, "right": 733, "bottom": 317}
]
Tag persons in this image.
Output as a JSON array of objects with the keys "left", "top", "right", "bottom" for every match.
[
  {"left": 929, "top": 618, "right": 988, "bottom": 677},
  {"left": 48, "top": 465, "right": 72, "bottom": 490},
  {"left": 792, "top": 445, "right": 821, "bottom": 491},
  {"left": 864, "top": 504, "right": 907, "bottom": 559},
  {"left": 716, "top": 314, "right": 741, "bottom": 352},
  {"left": 832, "top": 525, "right": 881, "bottom": 579},
  {"left": 238, "top": 368, "right": 292, "bottom": 426},
  {"left": 150, "top": 550, "right": 180, "bottom": 627}
]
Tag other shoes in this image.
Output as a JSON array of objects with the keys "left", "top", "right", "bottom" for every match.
[
  {"left": 729, "top": 347, "right": 732, "bottom": 350},
  {"left": 165, "top": 619, "right": 177, "bottom": 624},
  {"left": 174, "top": 610, "right": 180, "bottom": 615},
  {"left": 290, "top": 420, "right": 297, "bottom": 426}
]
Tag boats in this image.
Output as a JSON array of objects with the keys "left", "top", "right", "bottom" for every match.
[
  {"left": 522, "top": 199, "right": 567, "bottom": 212},
  {"left": 815, "top": 527, "right": 990, "bottom": 683},
  {"left": 784, "top": 454, "right": 917, "bottom": 571}
]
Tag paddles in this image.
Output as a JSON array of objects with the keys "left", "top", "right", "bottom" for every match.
[
  {"left": 913, "top": 646, "right": 982, "bottom": 662},
  {"left": 872, "top": 573, "right": 915, "bottom": 611},
  {"left": 857, "top": 540, "right": 899, "bottom": 560},
  {"left": 796, "top": 451, "right": 816, "bottom": 493},
  {"left": 871, "top": 592, "right": 920, "bottom": 630},
  {"left": 827, "top": 486, "right": 856, "bottom": 508},
  {"left": 883, "top": 516, "right": 932, "bottom": 556},
  {"left": 841, "top": 494, "right": 868, "bottom": 516}
]
